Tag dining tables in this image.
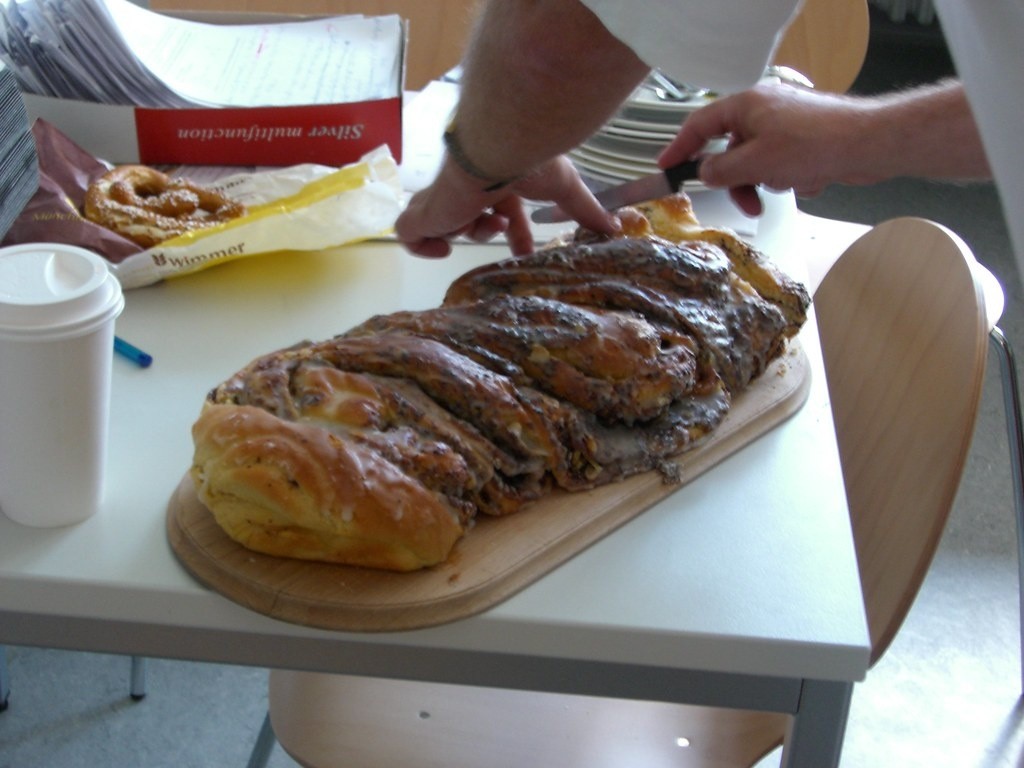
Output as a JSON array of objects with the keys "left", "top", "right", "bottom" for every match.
[{"left": 0, "top": 89, "right": 872, "bottom": 768}]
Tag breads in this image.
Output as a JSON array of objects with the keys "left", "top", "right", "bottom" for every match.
[
  {"left": 190, "top": 186, "right": 812, "bottom": 570},
  {"left": 85, "top": 162, "right": 245, "bottom": 246}
]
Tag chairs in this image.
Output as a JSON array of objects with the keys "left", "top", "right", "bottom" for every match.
[
  {"left": 142, "top": 0, "right": 484, "bottom": 93},
  {"left": 757, "top": 0, "right": 1024, "bottom": 698},
  {"left": 242, "top": 216, "right": 991, "bottom": 768}
]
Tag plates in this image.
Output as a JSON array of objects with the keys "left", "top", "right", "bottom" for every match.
[{"left": 561, "top": 74, "right": 717, "bottom": 190}]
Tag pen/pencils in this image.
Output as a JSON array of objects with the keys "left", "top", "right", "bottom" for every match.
[{"left": 114, "top": 335, "right": 152, "bottom": 368}]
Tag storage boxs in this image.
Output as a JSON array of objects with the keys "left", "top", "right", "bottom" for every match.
[{"left": 15, "top": 9, "right": 409, "bottom": 166}]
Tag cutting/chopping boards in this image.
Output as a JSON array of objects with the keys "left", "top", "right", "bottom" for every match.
[{"left": 162, "top": 342, "right": 810, "bottom": 631}]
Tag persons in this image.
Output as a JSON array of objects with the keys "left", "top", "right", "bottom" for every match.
[{"left": 394, "top": 0, "right": 1024, "bottom": 279}]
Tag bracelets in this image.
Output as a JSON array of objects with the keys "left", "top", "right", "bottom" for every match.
[{"left": 441, "top": 129, "right": 528, "bottom": 193}]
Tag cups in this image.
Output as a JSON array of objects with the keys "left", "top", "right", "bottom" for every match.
[{"left": 1, "top": 242, "right": 126, "bottom": 523}]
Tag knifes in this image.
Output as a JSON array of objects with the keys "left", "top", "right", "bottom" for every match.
[{"left": 532, "top": 162, "right": 700, "bottom": 222}]
